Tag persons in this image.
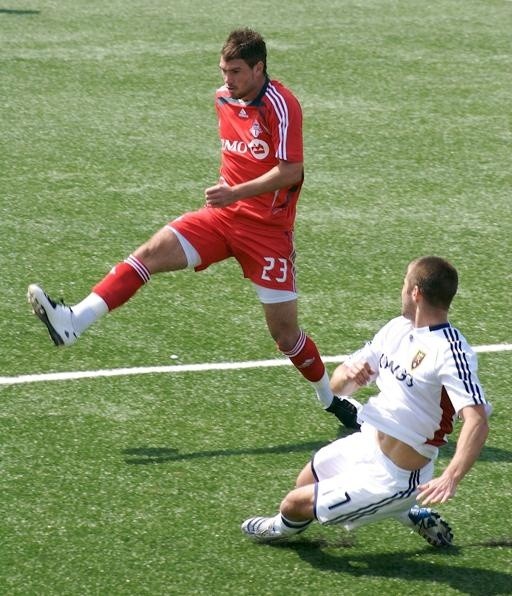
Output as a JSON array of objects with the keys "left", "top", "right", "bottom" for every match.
[
  {"left": 238, "top": 256, "right": 493, "bottom": 549},
  {"left": 27, "top": 28, "right": 365, "bottom": 429}
]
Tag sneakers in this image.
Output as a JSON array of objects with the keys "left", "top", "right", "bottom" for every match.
[
  {"left": 406, "top": 506, "right": 454, "bottom": 547},
  {"left": 326, "top": 396, "right": 360, "bottom": 429},
  {"left": 241, "top": 516, "right": 291, "bottom": 543},
  {"left": 29, "top": 284, "right": 78, "bottom": 347}
]
